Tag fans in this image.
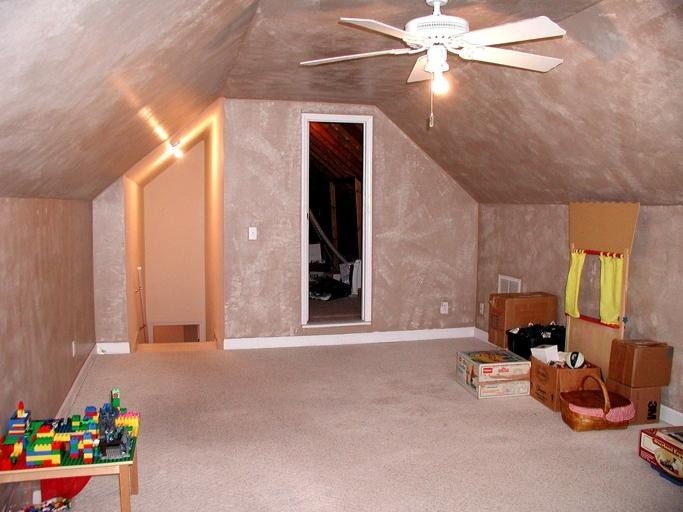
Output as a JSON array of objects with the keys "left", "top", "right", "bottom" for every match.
[{"left": 300, "top": 0, "right": 566, "bottom": 84}]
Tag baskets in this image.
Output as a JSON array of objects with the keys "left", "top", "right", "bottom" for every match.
[{"left": 557, "top": 372, "right": 635, "bottom": 432}]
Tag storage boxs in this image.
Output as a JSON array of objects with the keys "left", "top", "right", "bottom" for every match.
[
  {"left": 640, "top": 427, "right": 683, "bottom": 484},
  {"left": 455, "top": 349, "right": 531, "bottom": 399},
  {"left": 529, "top": 355, "right": 601, "bottom": 411},
  {"left": 605, "top": 377, "right": 661, "bottom": 425},
  {"left": 607, "top": 338, "right": 674, "bottom": 387}
]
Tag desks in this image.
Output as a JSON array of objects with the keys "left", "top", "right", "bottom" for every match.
[{"left": 0, "top": 411, "right": 143, "bottom": 512}]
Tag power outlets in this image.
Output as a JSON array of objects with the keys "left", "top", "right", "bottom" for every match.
[
  {"left": 440, "top": 301, "right": 448, "bottom": 314},
  {"left": 72, "top": 340, "right": 77, "bottom": 357},
  {"left": 479, "top": 303, "right": 484, "bottom": 316}
]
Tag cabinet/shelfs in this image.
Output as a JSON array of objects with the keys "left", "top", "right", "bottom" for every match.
[{"left": 487, "top": 293, "right": 558, "bottom": 351}]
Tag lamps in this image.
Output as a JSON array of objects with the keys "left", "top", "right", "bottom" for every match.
[{"left": 426, "top": 0, "right": 451, "bottom": 97}]
